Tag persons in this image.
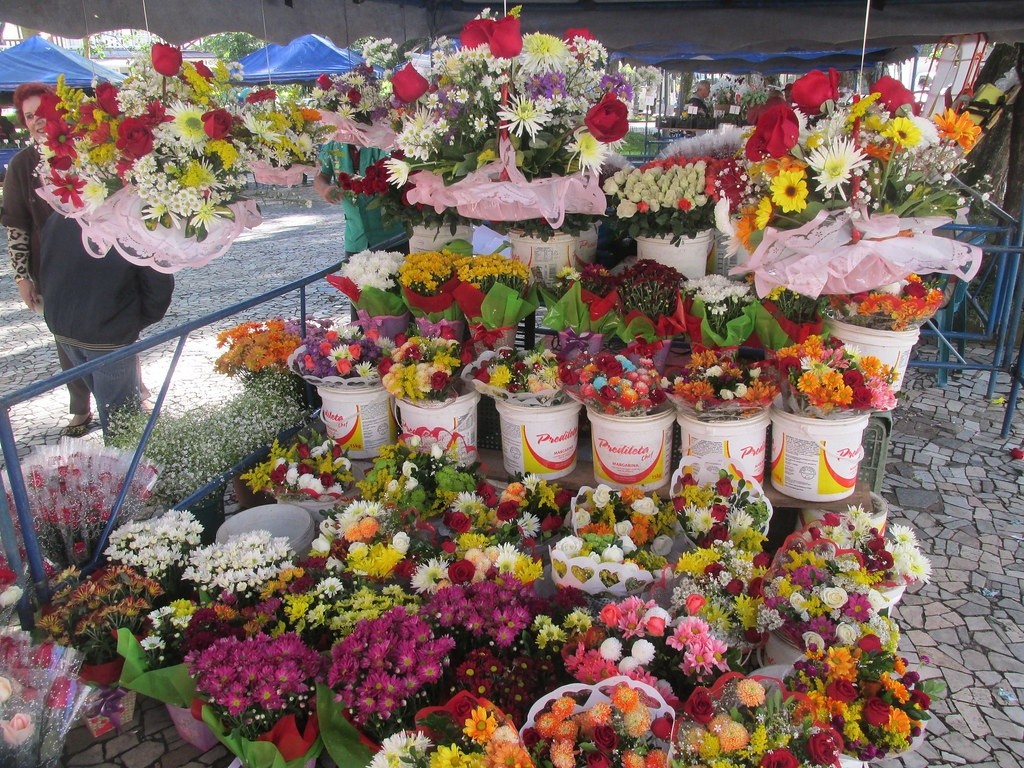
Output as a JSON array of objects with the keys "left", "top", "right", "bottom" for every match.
[
  {"left": 39, "top": 207, "right": 174, "bottom": 445},
  {"left": 313, "top": 129, "right": 412, "bottom": 328},
  {"left": 0, "top": 83, "right": 123, "bottom": 437},
  {"left": 684, "top": 79, "right": 712, "bottom": 118}
]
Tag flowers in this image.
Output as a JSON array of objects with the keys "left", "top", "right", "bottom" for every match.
[{"left": 0, "top": 4, "right": 1023, "bottom": 768}]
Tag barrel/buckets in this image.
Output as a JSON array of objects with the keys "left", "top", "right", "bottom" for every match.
[
  {"left": 771, "top": 394, "right": 871, "bottom": 502},
  {"left": 677, "top": 404, "right": 770, "bottom": 490},
  {"left": 408, "top": 218, "right": 790, "bottom": 289},
  {"left": 315, "top": 386, "right": 398, "bottom": 460},
  {"left": 274, "top": 465, "right": 368, "bottom": 524},
  {"left": 823, "top": 316, "right": 921, "bottom": 394},
  {"left": 395, "top": 378, "right": 480, "bottom": 468},
  {"left": 215, "top": 503, "right": 317, "bottom": 567},
  {"left": 495, "top": 400, "right": 582, "bottom": 480},
  {"left": 586, "top": 400, "right": 676, "bottom": 493}
]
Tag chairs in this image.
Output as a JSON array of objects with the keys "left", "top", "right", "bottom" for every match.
[{"left": 930, "top": 231, "right": 987, "bottom": 388}]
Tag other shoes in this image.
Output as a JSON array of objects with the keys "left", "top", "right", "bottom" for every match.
[{"left": 60, "top": 412, "right": 93, "bottom": 437}]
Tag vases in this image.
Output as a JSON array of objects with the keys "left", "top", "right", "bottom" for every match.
[
  {"left": 560, "top": 331, "right": 606, "bottom": 356},
  {"left": 761, "top": 346, "right": 793, "bottom": 375},
  {"left": 77, "top": 686, "right": 140, "bottom": 738},
  {"left": 414, "top": 315, "right": 464, "bottom": 343},
  {"left": 162, "top": 700, "right": 220, "bottom": 752},
  {"left": 687, "top": 341, "right": 739, "bottom": 365},
  {"left": 623, "top": 336, "right": 676, "bottom": 374},
  {"left": 78, "top": 653, "right": 130, "bottom": 689},
  {"left": 468, "top": 324, "right": 516, "bottom": 355},
  {"left": 350, "top": 310, "right": 408, "bottom": 341}
]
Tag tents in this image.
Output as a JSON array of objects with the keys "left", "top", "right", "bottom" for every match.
[
  {"left": 224, "top": 33, "right": 384, "bottom": 84},
  {"left": 598, "top": 43, "right": 920, "bottom": 78},
  {"left": 0, "top": 36, "right": 132, "bottom": 90}
]
